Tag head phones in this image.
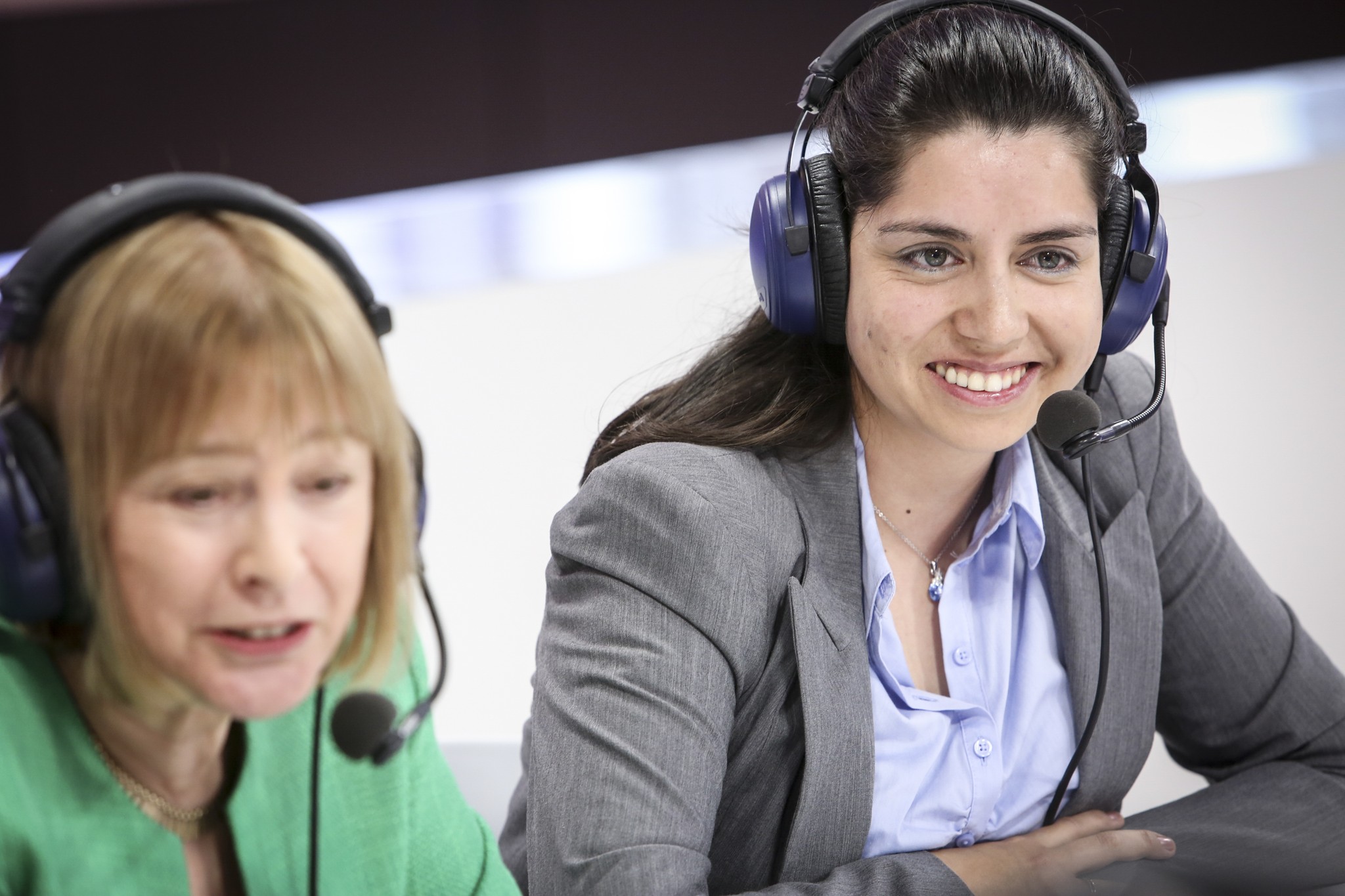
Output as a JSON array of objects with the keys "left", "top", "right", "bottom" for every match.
[
  {"left": 0, "top": 172, "right": 424, "bottom": 662},
  {"left": 749, "top": 0, "right": 1171, "bottom": 392}
]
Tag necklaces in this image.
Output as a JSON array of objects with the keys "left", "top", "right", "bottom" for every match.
[{"left": 872, "top": 497, "right": 982, "bottom": 603}]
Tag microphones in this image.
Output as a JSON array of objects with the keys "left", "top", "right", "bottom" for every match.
[
  {"left": 332, "top": 574, "right": 448, "bottom": 767},
  {"left": 1036, "top": 323, "right": 1167, "bottom": 460}
]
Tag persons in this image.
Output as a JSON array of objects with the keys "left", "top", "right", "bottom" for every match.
[
  {"left": 498, "top": 0, "right": 1345, "bottom": 896},
  {"left": 0, "top": 182, "right": 521, "bottom": 896}
]
